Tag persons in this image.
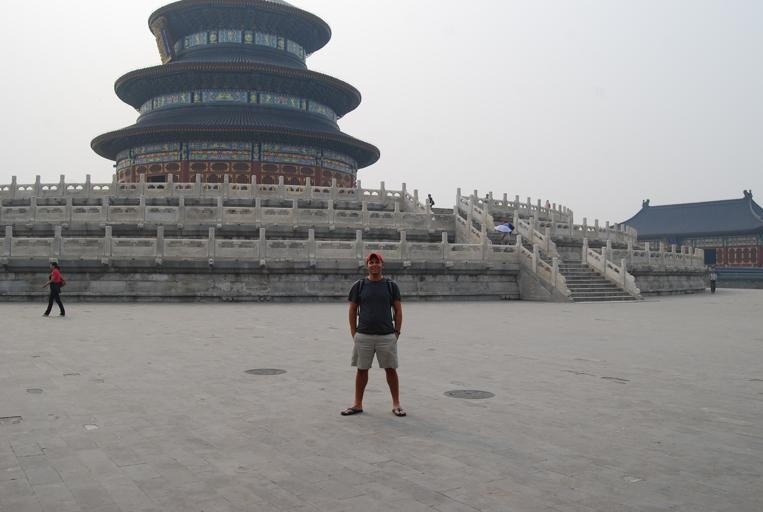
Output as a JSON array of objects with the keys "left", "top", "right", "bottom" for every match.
[
  {"left": 709, "top": 269, "right": 717, "bottom": 293},
  {"left": 503, "top": 219, "right": 511, "bottom": 235},
  {"left": 341, "top": 252, "right": 407, "bottom": 416},
  {"left": 501, "top": 233, "right": 510, "bottom": 245},
  {"left": 42, "top": 262, "right": 66, "bottom": 317},
  {"left": 428, "top": 194, "right": 434, "bottom": 210}
]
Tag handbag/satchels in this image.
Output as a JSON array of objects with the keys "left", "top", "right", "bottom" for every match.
[{"left": 60, "top": 277, "right": 65, "bottom": 288}]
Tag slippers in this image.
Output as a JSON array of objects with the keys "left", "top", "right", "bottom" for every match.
[
  {"left": 341, "top": 407, "right": 363, "bottom": 416},
  {"left": 392, "top": 408, "right": 407, "bottom": 416}
]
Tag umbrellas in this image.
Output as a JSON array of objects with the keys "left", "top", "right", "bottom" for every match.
[{"left": 494, "top": 224, "right": 512, "bottom": 232}]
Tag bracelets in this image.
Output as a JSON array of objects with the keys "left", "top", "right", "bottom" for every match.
[{"left": 394, "top": 329, "right": 400, "bottom": 334}]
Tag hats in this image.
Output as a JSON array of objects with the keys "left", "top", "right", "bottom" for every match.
[{"left": 366, "top": 253, "right": 385, "bottom": 265}]
